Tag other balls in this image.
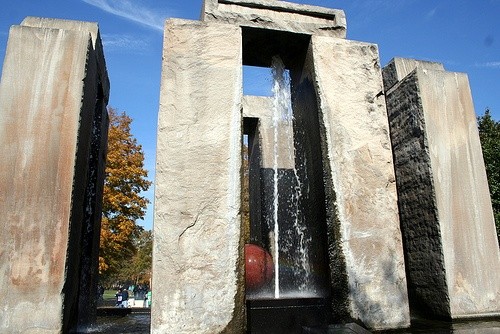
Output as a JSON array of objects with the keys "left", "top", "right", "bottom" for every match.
[{"left": 245, "top": 243, "right": 274, "bottom": 294}]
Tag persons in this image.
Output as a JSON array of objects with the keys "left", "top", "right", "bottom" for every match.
[
  {"left": 99, "top": 283, "right": 104, "bottom": 296},
  {"left": 114, "top": 281, "right": 151, "bottom": 309}
]
